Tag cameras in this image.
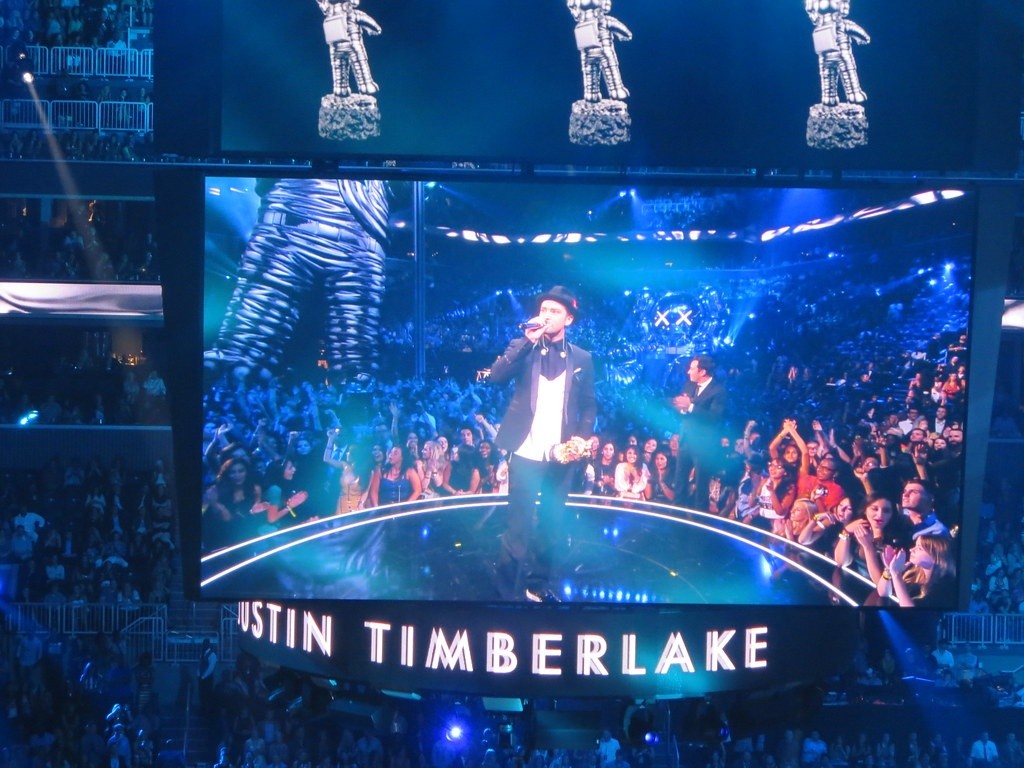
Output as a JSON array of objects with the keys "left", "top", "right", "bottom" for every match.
[{"left": 816, "top": 487, "right": 827, "bottom": 496}]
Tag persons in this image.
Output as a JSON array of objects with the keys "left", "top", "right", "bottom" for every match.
[
  {"left": 0, "top": 0, "right": 190, "bottom": 768},
  {"left": 970, "top": 384, "right": 1024, "bottom": 614},
  {"left": 202, "top": 278, "right": 969, "bottom": 607},
  {"left": 196, "top": 639, "right": 1024, "bottom": 768},
  {"left": 672, "top": 354, "right": 725, "bottom": 514},
  {"left": 489, "top": 285, "right": 596, "bottom": 603}
]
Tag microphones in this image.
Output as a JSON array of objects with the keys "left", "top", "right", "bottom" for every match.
[{"left": 518, "top": 322, "right": 547, "bottom": 330}]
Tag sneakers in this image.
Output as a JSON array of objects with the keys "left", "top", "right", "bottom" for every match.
[{"left": 525, "top": 587, "right": 561, "bottom": 602}]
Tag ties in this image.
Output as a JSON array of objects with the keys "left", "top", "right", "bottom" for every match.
[{"left": 692, "top": 384, "right": 702, "bottom": 406}]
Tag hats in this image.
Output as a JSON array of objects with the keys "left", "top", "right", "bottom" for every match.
[{"left": 535, "top": 285, "right": 578, "bottom": 325}]
